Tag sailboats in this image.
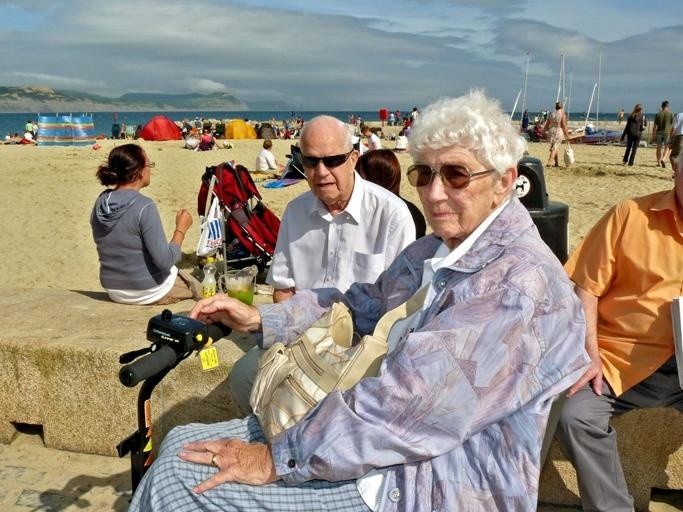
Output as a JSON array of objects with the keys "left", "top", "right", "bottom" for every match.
[
  {"left": 508, "top": 47, "right": 544, "bottom": 142},
  {"left": 581, "top": 45, "right": 624, "bottom": 143},
  {"left": 539, "top": 47, "right": 586, "bottom": 145}
]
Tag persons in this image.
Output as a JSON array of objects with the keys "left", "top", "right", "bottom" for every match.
[
  {"left": 354, "top": 149, "right": 426, "bottom": 241},
  {"left": 128, "top": 87, "right": 594, "bottom": 512},
  {"left": 90, "top": 144, "right": 201, "bottom": 305},
  {"left": 230, "top": 114, "right": 416, "bottom": 418},
  {"left": 560, "top": 134, "right": 683, "bottom": 512}
]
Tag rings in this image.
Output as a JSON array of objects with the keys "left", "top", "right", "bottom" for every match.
[{"left": 211, "top": 455, "right": 216, "bottom": 468}]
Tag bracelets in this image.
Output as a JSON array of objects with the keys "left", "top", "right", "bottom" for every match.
[{"left": 173, "top": 229, "right": 184, "bottom": 239}]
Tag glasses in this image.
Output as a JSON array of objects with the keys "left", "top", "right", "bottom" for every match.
[
  {"left": 301, "top": 148, "right": 354, "bottom": 168},
  {"left": 145, "top": 161, "right": 155, "bottom": 168},
  {"left": 407, "top": 165, "right": 498, "bottom": 188}
]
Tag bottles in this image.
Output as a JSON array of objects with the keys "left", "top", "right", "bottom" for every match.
[
  {"left": 200, "top": 256, "right": 217, "bottom": 300},
  {"left": 229, "top": 199, "right": 248, "bottom": 227}
]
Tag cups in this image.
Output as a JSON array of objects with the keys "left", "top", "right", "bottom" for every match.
[{"left": 225, "top": 275, "right": 255, "bottom": 308}]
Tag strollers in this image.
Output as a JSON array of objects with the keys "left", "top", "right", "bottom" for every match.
[
  {"left": 196, "top": 162, "right": 276, "bottom": 275},
  {"left": 229, "top": 160, "right": 280, "bottom": 240}
]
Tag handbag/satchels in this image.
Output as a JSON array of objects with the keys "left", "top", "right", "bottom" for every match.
[
  {"left": 250, "top": 302, "right": 389, "bottom": 442},
  {"left": 564, "top": 147, "right": 575, "bottom": 167},
  {"left": 196, "top": 198, "right": 222, "bottom": 256}
]
